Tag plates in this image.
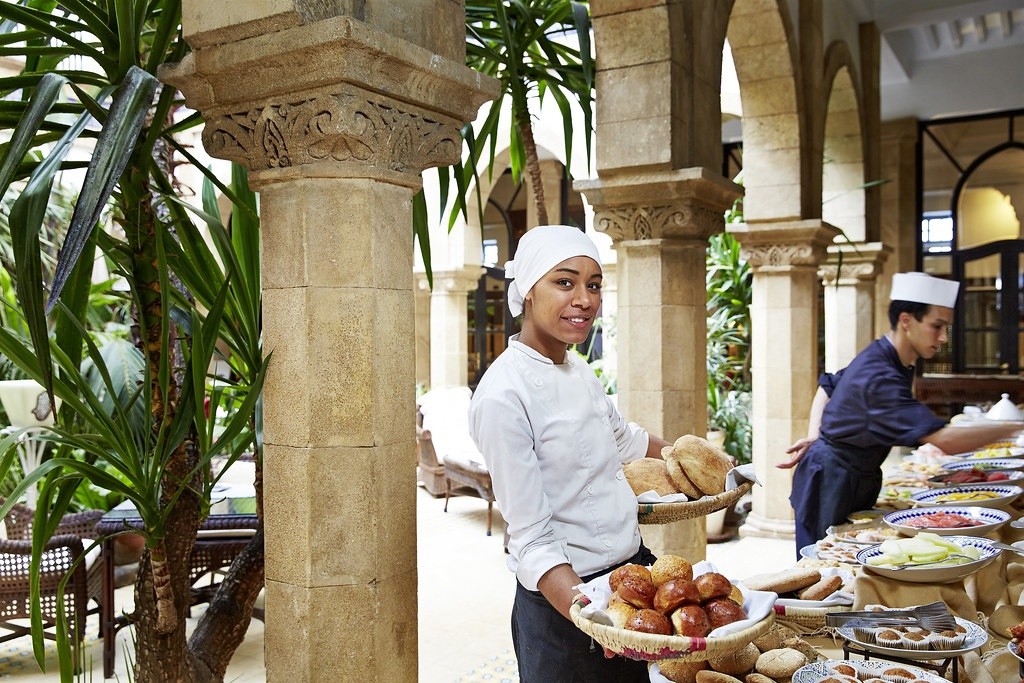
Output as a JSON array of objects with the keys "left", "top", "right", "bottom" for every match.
[
  {"left": 791, "top": 659, "right": 953, "bottom": 683},
  {"left": 794, "top": 391, "right": 1024, "bottom": 572},
  {"left": 1007, "top": 640, "right": 1024, "bottom": 662},
  {"left": 832, "top": 607, "right": 989, "bottom": 659},
  {"left": 855, "top": 533, "right": 1005, "bottom": 585}
]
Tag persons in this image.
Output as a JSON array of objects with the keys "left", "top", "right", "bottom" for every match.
[
  {"left": 468, "top": 224, "right": 683, "bottom": 683},
  {"left": 777, "top": 271, "right": 1022, "bottom": 570}
]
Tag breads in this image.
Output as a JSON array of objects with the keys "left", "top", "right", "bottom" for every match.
[{"left": 603, "top": 553, "right": 746, "bottom": 637}]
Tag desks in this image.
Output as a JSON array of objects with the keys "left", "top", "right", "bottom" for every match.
[
  {"left": 97, "top": 495, "right": 264, "bottom": 679},
  {"left": 671, "top": 395, "right": 1023, "bottom": 683}
]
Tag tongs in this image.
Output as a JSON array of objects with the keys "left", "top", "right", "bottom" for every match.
[{"left": 825, "top": 601, "right": 956, "bottom": 634}]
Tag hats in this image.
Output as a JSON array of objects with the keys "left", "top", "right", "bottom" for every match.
[{"left": 889, "top": 271, "right": 961, "bottom": 309}]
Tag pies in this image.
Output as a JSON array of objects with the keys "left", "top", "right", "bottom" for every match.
[
  {"left": 623, "top": 434, "right": 734, "bottom": 500},
  {"left": 657, "top": 569, "right": 843, "bottom": 683}
]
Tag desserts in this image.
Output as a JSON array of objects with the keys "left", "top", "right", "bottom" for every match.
[{"left": 816, "top": 606, "right": 967, "bottom": 683}]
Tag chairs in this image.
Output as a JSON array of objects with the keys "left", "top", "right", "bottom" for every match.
[
  {"left": 0, "top": 495, "right": 111, "bottom": 677},
  {"left": 413, "top": 382, "right": 472, "bottom": 499}
]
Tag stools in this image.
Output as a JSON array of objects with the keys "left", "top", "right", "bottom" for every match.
[{"left": 442, "top": 444, "right": 500, "bottom": 533}]
